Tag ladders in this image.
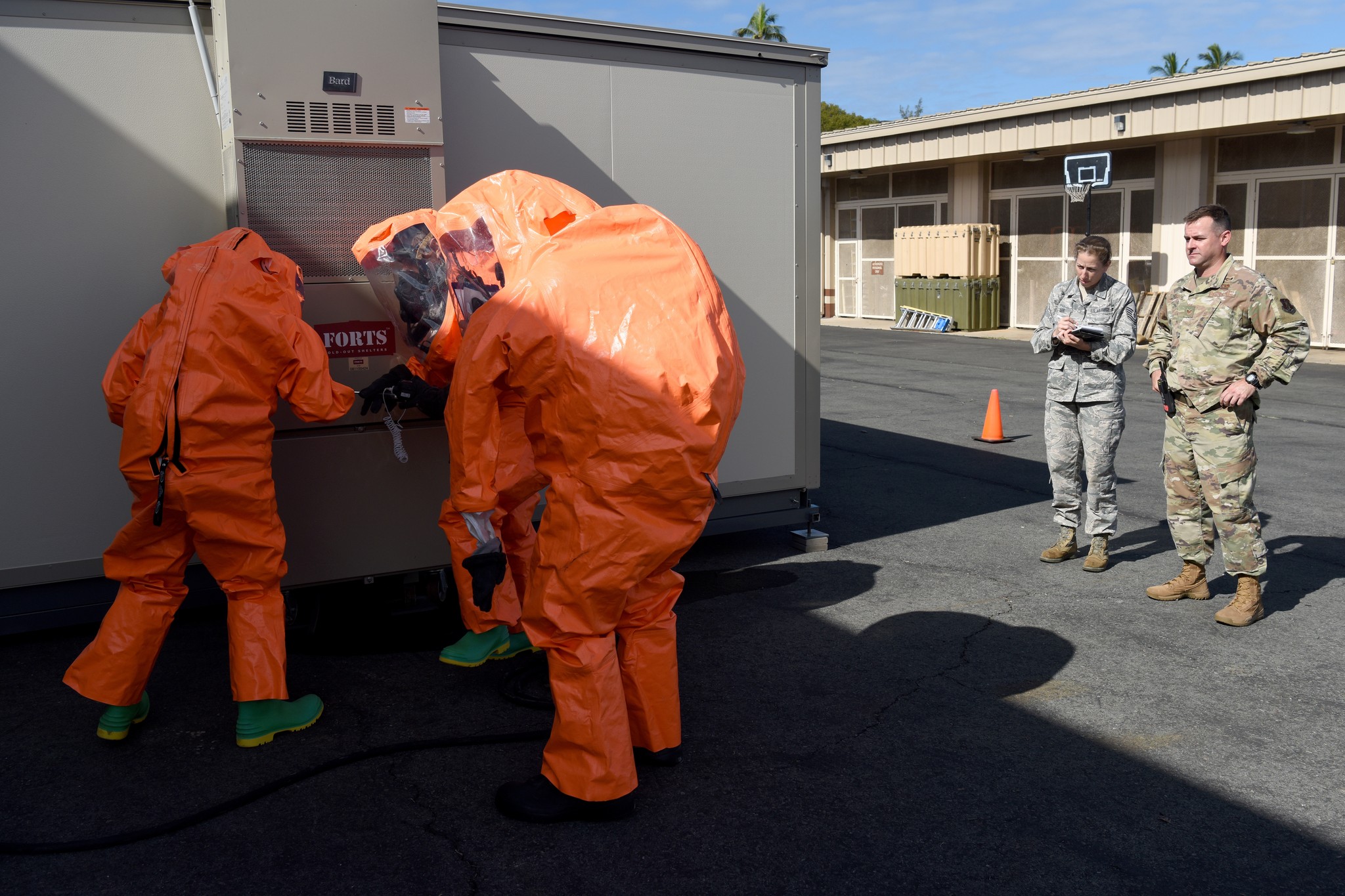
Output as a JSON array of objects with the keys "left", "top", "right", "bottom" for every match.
[{"left": 890, "top": 305, "right": 954, "bottom": 333}]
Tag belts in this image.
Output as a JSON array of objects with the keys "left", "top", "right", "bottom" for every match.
[{"left": 1172, "top": 390, "right": 1194, "bottom": 407}]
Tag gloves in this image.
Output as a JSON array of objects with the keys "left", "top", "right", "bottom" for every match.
[
  {"left": 392, "top": 375, "right": 432, "bottom": 409},
  {"left": 462, "top": 537, "right": 507, "bottom": 612},
  {"left": 360, "top": 363, "right": 413, "bottom": 415}
]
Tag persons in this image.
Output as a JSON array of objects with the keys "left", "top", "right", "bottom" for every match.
[
  {"left": 1143, "top": 204, "right": 1310, "bottom": 626},
  {"left": 1030, "top": 236, "right": 1137, "bottom": 572},
  {"left": 355, "top": 169, "right": 746, "bottom": 818},
  {"left": 62, "top": 229, "right": 356, "bottom": 746}
]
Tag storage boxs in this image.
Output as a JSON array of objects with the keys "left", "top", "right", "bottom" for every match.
[{"left": 893, "top": 223, "right": 1001, "bottom": 332}]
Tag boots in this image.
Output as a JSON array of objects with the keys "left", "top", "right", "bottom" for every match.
[
  {"left": 1082, "top": 532, "right": 1110, "bottom": 572},
  {"left": 1215, "top": 573, "right": 1264, "bottom": 627},
  {"left": 1040, "top": 525, "right": 1078, "bottom": 563},
  {"left": 1147, "top": 560, "right": 1210, "bottom": 601}
]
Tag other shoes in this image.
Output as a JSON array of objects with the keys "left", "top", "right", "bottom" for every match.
[
  {"left": 495, "top": 775, "right": 637, "bottom": 824},
  {"left": 439, "top": 624, "right": 510, "bottom": 667},
  {"left": 488, "top": 631, "right": 542, "bottom": 660},
  {"left": 95, "top": 689, "right": 150, "bottom": 740},
  {"left": 235, "top": 695, "right": 325, "bottom": 747},
  {"left": 633, "top": 745, "right": 678, "bottom": 773}
]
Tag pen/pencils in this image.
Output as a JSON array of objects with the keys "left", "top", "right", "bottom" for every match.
[{"left": 1057, "top": 315, "right": 1078, "bottom": 327}]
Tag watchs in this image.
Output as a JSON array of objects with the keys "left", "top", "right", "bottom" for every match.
[{"left": 1245, "top": 372, "right": 1263, "bottom": 390}]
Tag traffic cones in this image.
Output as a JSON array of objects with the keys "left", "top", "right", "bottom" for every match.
[{"left": 970, "top": 389, "right": 1014, "bottom": 443}]
